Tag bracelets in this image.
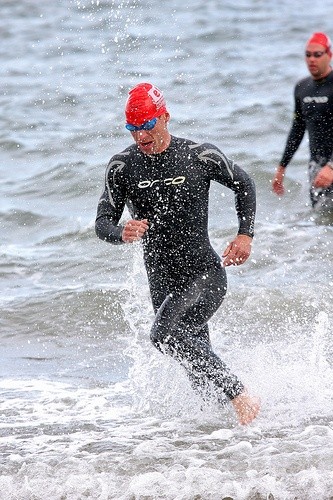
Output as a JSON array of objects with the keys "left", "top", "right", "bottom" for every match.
[{"left": 327, "top": 162, "right": 333, "bottom": 170}]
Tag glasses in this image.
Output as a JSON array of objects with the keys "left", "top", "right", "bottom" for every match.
[
  {"left": 305, "top": 42, "right": 332, "bottom": 58},
  {"left": 125, "top": 115, "right": 163, "bottom": 131}
]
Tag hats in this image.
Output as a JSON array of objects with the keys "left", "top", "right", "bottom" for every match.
[
  {"left": 125, "top": 82, "right": 167, "bottom": 127},
  {"left": 306, "top": 32, "right": 333, "bottom": 58},
  {"left": 125, "top": 83, "right": 166, "bottom": 126}
]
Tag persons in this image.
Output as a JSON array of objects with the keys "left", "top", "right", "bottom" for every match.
[
  {"left": 95, "top": 82, "right": 257, "bottom": 426},
  {"left": 272, "top": 32, "right": 333, "bottom": 210}
]
